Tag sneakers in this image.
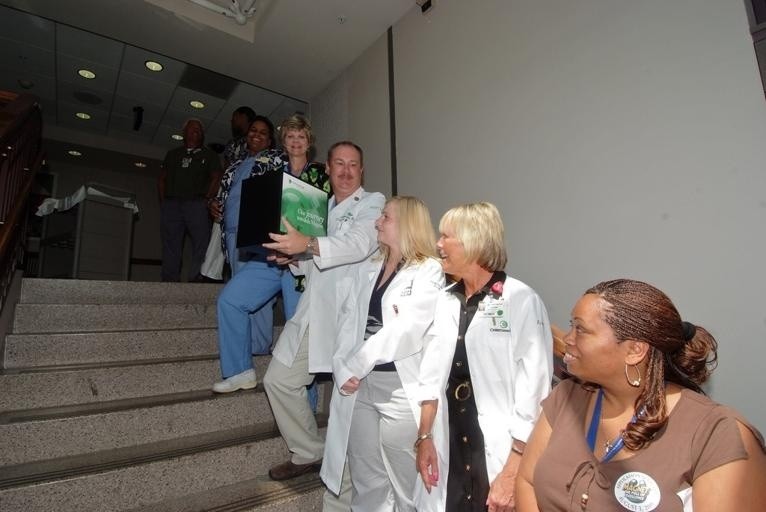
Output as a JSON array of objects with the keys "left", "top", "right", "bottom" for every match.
[{"left": 213, "top": 368, "right": 257, "bottom": 393}]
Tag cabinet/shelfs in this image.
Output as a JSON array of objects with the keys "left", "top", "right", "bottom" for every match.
[{"left": 36, "top": 184, "right": 135, "bottom": 282}]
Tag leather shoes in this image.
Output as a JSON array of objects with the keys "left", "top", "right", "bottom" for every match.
[
  {"left": 269, "top": 461, "right": 321, "bottom": 480},
  {"left": 194, "top": 274, "right": 223, "bottom": 283}
]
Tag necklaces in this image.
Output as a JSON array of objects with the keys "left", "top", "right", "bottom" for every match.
[{"left": 597, "top": 425, "right": 624, "bottom": 456}]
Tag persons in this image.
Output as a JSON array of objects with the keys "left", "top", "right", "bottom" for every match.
[
  {"left": 513, "top": 279, "right": 765, "bottom": 512},
  {"left": 261, "top": 140, "right": 388, "bottom": 512},
  {"left": 204, "top": 115, "right": 287, "bottom": 356},
  {"left": 197, "top": 106, "right": 256, "bottom": 278},
  {"left": 154, "top": 116, "right": 212, "bottom": 283},
  {"left": 210, "top": 112, "right": 333, "bottom": 417},
  {"left": 412, "top": 200, "right": 553, "bottom": 511},
  {"left": 317, "top": 197, "right": 447, "bottom": 511}
]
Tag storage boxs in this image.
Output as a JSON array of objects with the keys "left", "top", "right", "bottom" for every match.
[{"left": 235, "top": 170, "right": 329, "bottom": 266}]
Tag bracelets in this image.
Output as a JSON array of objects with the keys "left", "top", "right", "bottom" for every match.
[
  {"left": 510, "top": 446, "right": 525, "bottom": 456},
  {"left": 413, "top": 431, "right": 432, "bottom": 446},
  {"left": 205, "top": 196, "right": 219, "bottom": 209},
  {"left": 305, "top": 235, "right": 316, "bottom": 255}
]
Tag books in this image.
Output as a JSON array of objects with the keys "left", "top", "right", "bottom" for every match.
[{"left": 234, "top": 171, "right": 327, "bottom": 248}]
